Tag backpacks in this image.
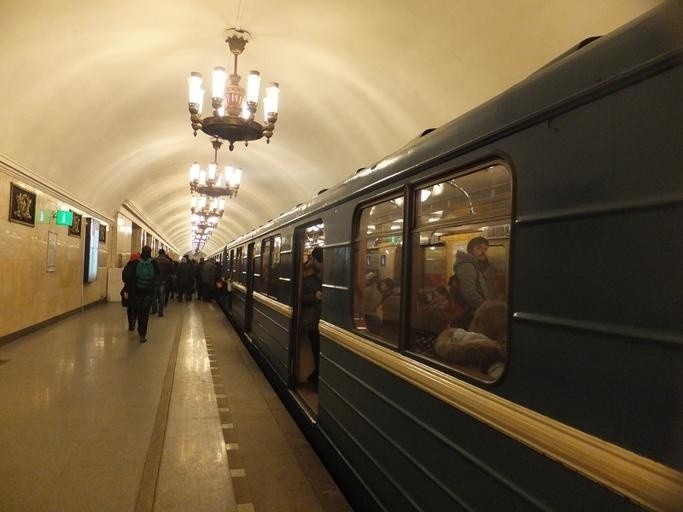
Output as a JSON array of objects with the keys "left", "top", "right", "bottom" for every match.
[
  {"left": 447, "top": 265, "right": 485, "bottom": 321},
  {"left": 136, "top": 257, "right": 157, "bottom": 292}
]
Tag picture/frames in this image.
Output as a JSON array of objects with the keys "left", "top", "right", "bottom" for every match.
[
  {"left": 8, "top": 181, "right": 38, "bottom": 228},
  {"left": 99, "top": 224, "right": 106, "bottom": 242},
  {"left": 68, "top": 208, "right": 82, "bottom": 238}
]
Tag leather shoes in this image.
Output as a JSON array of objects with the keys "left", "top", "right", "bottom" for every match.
[{"left": 169, "top": 295, "right": 212, "bottom": 303}]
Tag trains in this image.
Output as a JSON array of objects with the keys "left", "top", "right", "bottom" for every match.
[{"left": 204, "top": 0, "right": 683, "bottom": 512}]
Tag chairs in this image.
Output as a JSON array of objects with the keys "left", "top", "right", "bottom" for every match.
[{"left": 361, "top": 287, "right": 508, "bottom": 368}]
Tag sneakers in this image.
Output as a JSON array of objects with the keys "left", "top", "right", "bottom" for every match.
[
  {"left": 306, "top": 373, "right": 319, "bottom": 393},
  {"left": 151, "top": 311, "right": 157, "bottom": 314},
  {"left": 140, "top": 337, "right": 147, "bottom": 343},
  {"left": 128, "top": 316, "right": 136, "bottom": 331}
]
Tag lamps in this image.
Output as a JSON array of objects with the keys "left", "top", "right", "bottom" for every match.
[
  {"left": 190, "top": 140, "right": 243, "bottom": 256},
  {"left": 53, "top": 209, "right": 73, "bottom": 227},
  {"left": 186, "top": 27, "right": 280, "bottom": 155}
]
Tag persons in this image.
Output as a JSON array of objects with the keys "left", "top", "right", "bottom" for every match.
[
  {"left": 151, "top": 248, "right": 169, "bottom": 318},
  {"left": 364, "top": 260, "right": 508, "bottom": 384},
  {"left": 157, "top": 254, "right": 221, "bottom": 307},
  {"left": 453, "top": 236, "right": 497, "bottom": 332},
  {"left": 127, "top": 246, "right": 159, "bottom": 342},
  {"left": 14, "top": 192, "right": 30, "bottom": 220},
  {"left": 121, "top": 251, "right": 139, "bottom": 331},
  {"left": 300, "top": 247, "right": 323, "bottom": 392}
]
